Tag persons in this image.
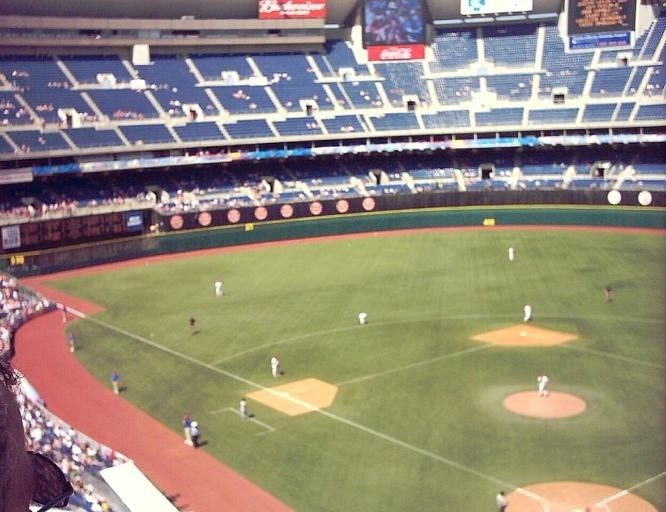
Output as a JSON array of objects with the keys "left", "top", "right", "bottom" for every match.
[
  {"left": 403, "top": 156, "right": 645, "bottom": 193},
  {"left": 538, "top": 371, "right": 549, "bottom": 398},
  {"left": 239, "top": 398, "right": 248, "bottom": 422},
  {"left": 508, "top": 247, "right": 515, "bottom": 262},
  {"left": 359, "top": 312, "right": 368, "bottom": 325},
  {"left": 523, "top": 303, "right": 532, "bottom": 322},
  {"left": 188, "top": 317, "right": 196, "bottom": 335},
  {"left": 270, "top": 354, "right": 281, "bottom": 377},
  {"left": 215, "top": 279, "right": 223, "bottom": 296},
  {"left": 604, "top": 286, "right": 615, "bottom": 305},
  {"left": 364, "top": 0, "right": 424, "bottom": 46},
  {"left": 183, "top": 411, "right": 194, "bottom": 446},
  {"left": 0, "top": 272, "right": 127, "bottom": 512},
  {"left": 455, "top": 56, "right": 659, "bottom": 99},
  {"left": 495, "top": 490, "right": 508, "bottom": 512},
  {"left": 190, "top": 420, "right": 199, "bottom": 448},
  {"left": 2, "top": 147, "right": 401, "bottom": 226},
  {"left": 0, "top": 70, "right": 182, "bottom": 154},
  {"left": 206, "top": 66, "right": 397, "bottom": 133}
]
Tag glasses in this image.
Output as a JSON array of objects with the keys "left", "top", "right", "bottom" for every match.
[{"left": 23, "top": 449, "right": 74, "bottom": 512}]
